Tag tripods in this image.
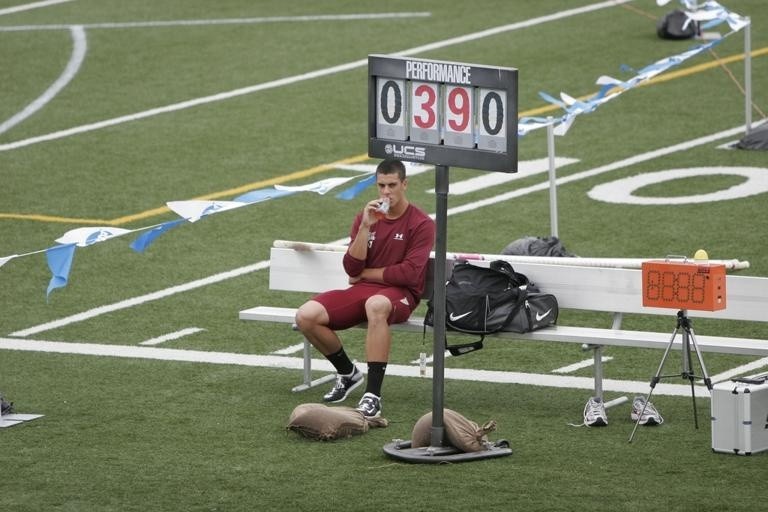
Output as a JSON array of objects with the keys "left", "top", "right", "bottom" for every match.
[{"left": 629, "top": 311, "right": 713, "bottom": 444}]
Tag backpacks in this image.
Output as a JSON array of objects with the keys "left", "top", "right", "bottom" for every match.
[{"left": 423, "top": 260, "right": 558, "bottom": 334}]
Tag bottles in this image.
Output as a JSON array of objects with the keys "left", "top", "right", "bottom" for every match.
[{"left": 373, "top": 197, "right": 391, "bottom": 219}]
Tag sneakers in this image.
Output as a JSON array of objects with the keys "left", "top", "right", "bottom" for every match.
[
  {"left": 323, "top": 364, "right": 365, "bottom": 404},
  {"left": 631, "top": 396, "right": 663, "bottom": 426},
  {"left": 583, "top": 395, "right": 609, "bottom": 426},
  {"left": 355, "top": 392, "right": 381, "bottom": 420}
]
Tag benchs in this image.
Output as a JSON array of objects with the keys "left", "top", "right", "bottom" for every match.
[{"left": 239, "top": 240, "right": 766, "bottom": 403}]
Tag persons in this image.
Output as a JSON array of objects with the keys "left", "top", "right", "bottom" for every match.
[{"left": 294, "top": 158, "right": 436, "bottom": 420}]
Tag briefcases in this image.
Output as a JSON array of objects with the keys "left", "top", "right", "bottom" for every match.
[{"left": 710, "top": 371, "right": 768, "bottom": 454}]
